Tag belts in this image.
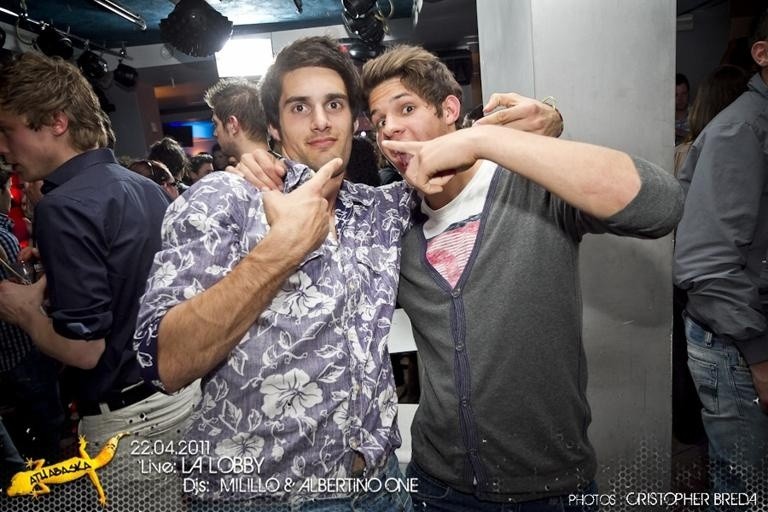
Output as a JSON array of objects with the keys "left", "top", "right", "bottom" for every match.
[{"left": 74, "top": 379, "right": 160, "bottom": 420}]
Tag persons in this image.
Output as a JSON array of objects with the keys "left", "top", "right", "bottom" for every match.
[
  {"left": 670, "top": 61, "right": 749, "bottom": 181},
  {"left": 1, "top": 48, "right": 174, "bottom": 464},
  {"left": 202, "top": 79, "right": 286, "bottom": 176},
  {"left": 667, "top": 7, "right": 767, "bottom": 512},
  {"left": 0, "top": 105, "right": 482, "bottom": 496},
  {"left": 676, "top": 72, "right": 697, "bottom": 148},
  {"left": 129, "top": 34, "right": 565, "bottom": 509},
  {"left": 221, "top": 42, "right": 688, "bottom": 509}
]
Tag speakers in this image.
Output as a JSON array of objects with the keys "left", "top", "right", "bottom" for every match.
[{"left": 162, "top": 125, "right": 193, "bottom": 147}]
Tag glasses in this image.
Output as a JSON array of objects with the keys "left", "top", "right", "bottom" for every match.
[{"left": 168, "top": 180, "right": 180, "bottom": 187}]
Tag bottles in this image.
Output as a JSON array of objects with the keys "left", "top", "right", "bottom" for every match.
[{"left": 8, "top": 240, "right": 46, "bottom": 303}]
